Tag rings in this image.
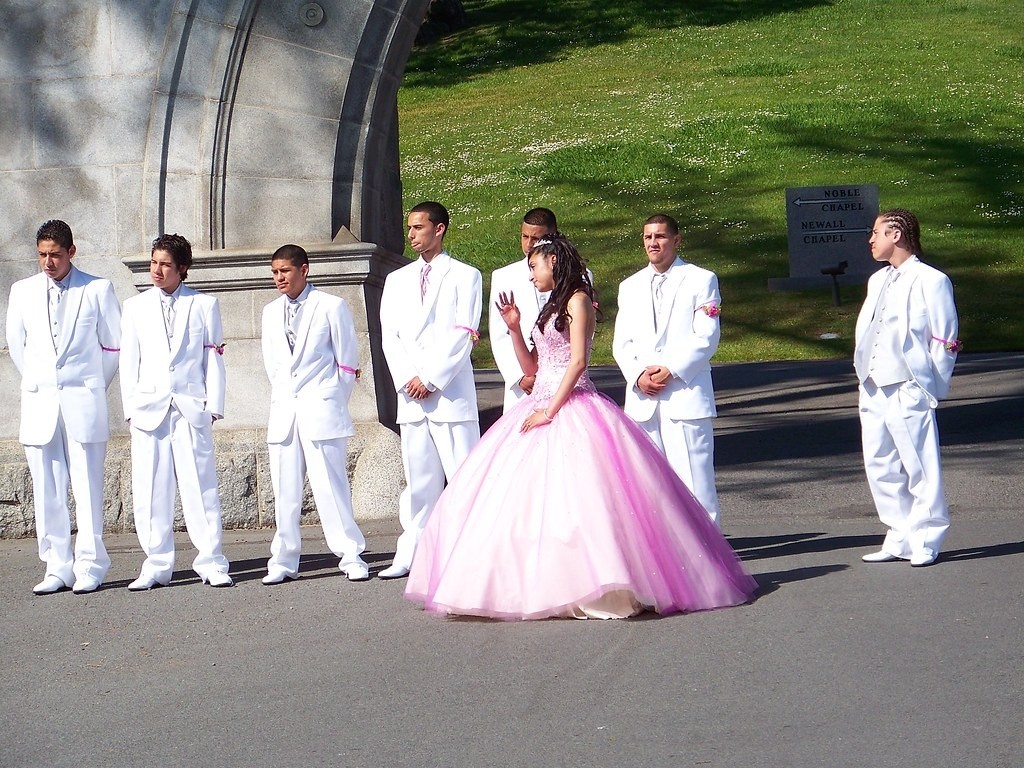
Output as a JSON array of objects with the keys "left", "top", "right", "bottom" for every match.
[
  {"left": 501, "top": 304, "right": 505, "bottom": 306},
  {"left": 526, "top": 424, "right": 530, "bottom": 427}
]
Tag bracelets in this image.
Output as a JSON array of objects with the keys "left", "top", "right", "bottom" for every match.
[{"left": 543, "top": 408, "right": 552, "bottom": 421}]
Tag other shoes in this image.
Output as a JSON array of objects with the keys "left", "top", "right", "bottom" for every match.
[
  {"left": 377, "top": 565, "right": 410, "bottom": 578},
  {"left": 911, "top": 553, "right": 935, "bottom": 567},
  {"left": 127, "top": 574, "right": 159, "bottom": 591},
  {"left": 261, "top": 572, "right": 290, "bottom": 585},
  {"left": 346, "top": 564, "right": 369, "bottom": 581},
  {"left": 861, "top": 549, "right": 909, "bottom": 562},
  {"left": 71, "top": 577, "right": 100, "bottom": 593},
  {"left": 206, "top": 571, "right": 232, "bottom": 587},
  {"left": 32, "top": 575, "right": 65, "bottom": 594}
]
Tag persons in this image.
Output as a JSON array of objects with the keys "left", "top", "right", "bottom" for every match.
[
  {"left": 853, "top": 209, "right": 962, "bottom": 567},
  {"left": 613, "top": 214, "right": 721, "bottom": 530},
  {"left": 6, "top": 219, "right": 121, "bottom": 595},
  {"left": 488, "top": 207, "right": 593, "bottom": 414},
  {"left": 119, "top": 233, "right": 234, "bottom": 591},
  {"left": 403, "top": 231, "right": 759, "bottom": 621},
  {"left": 261, "top": 244, "right": 369, "bottom": 585},
  {"left": 377, "top": 201, "right": 482, "bottom": 579}
]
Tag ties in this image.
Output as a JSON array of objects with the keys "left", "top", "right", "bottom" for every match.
[
  {"left": 655, "top": 274, "right": 668, "bottom": 304},
  {"left": 888, "top": 269, "right": 901, "bottom": 287},
  {"left": 289, "top": 302, "right": 302, "bottom": 326},
  {"left": 51, "top": 284, "right": 64, "bottom": 306},
  {"left": 162, "top": 296, "right": 176, "bottom": 326},
  {"left": 420, "top": 264, "right": 431, "bottom": 305}
]
